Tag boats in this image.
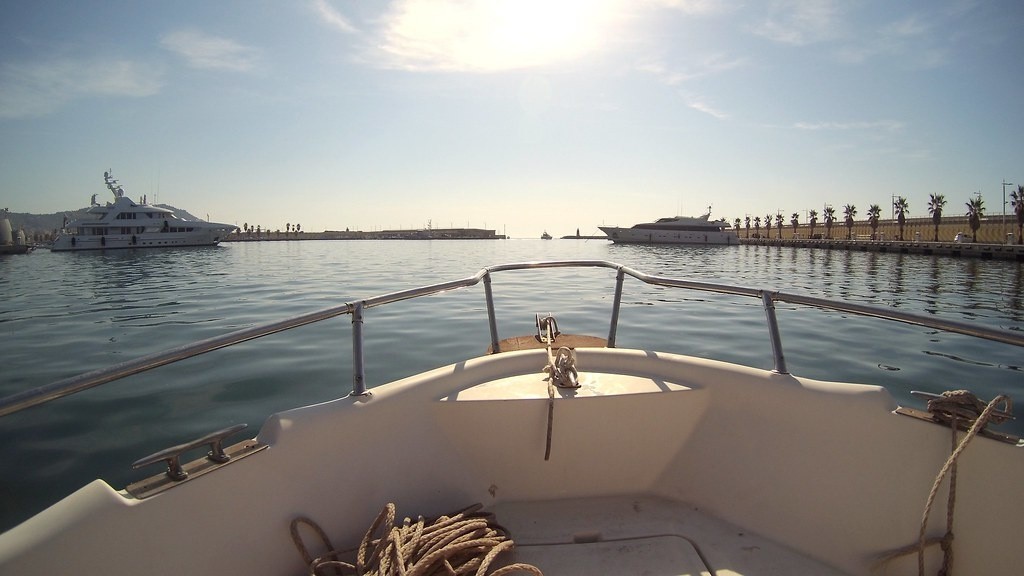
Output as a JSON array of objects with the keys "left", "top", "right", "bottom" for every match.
[
  {"left": 597, "top": 205, "right": 743, "bottom": 246},
  {"left": 43, "top": 168, "right": 239, "bottom": 253},
  {"left": 540, "top": 229, "right": 552, "bottom": 240}
]
